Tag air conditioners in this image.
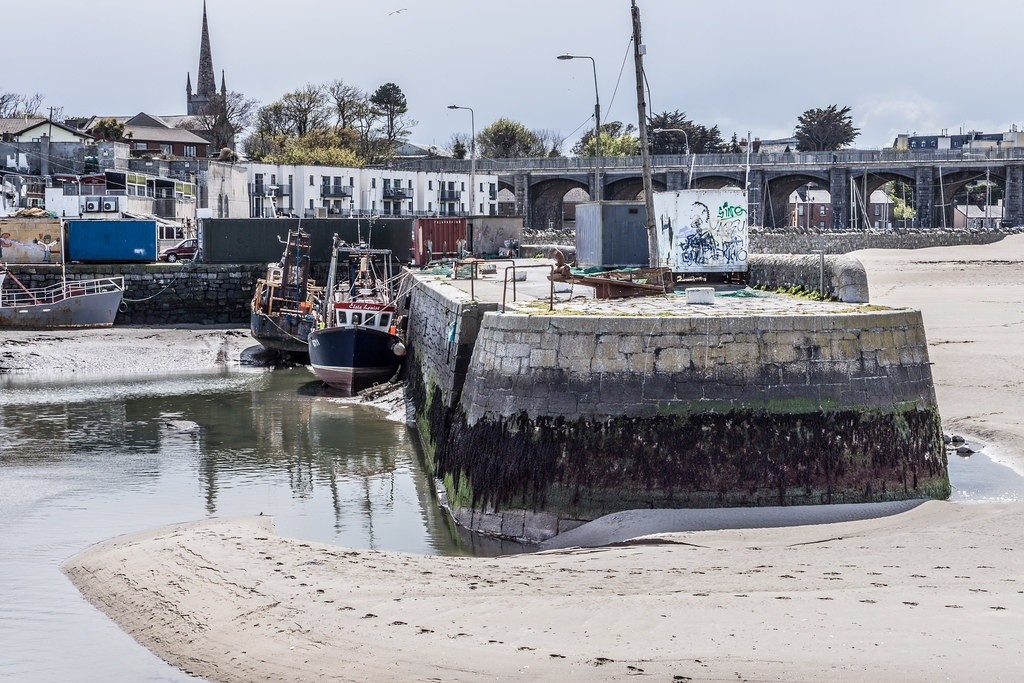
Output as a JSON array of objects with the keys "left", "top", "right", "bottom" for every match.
[
  {"left": 87, "top": 201, "right": 99, "bottom": 211},
  {"left": 103, "top": 201, "right": 115, "bottom": 212}
]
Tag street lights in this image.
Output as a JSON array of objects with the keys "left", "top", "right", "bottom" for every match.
[
  {"left": 652, "top": 128, "right": 692, "bottom": 186},
  {"left": 963, "top": 152, "right": 988, "bottom": 224},
  {"left": 448, "top": 104, "right": 475, "bottom": 214},
  {"left": 557, "top": 53, "right": 600, "bottom": 198}
]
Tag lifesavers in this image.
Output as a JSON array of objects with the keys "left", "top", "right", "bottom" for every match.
[{"left": 118, "top": 302, "right": 128, "bottom": 313}]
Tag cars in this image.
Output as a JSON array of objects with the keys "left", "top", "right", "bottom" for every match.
[{"left": 159, "top": 238, "right": 197, "bottom": 263}]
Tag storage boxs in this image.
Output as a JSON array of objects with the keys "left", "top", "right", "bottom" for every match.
[
  {"left": 196, "top": 218, "right": 413, "bottom": 264},
  {"left": 511, "top": 270, "right": 527, "bottom": 280},
  {"left": 686, "top": 288, "right": 716, "bottom": 303},
  {"left": 66, "top": 220, "right": 159, "bottom": 264},
  {"left": 414, "top": 218, "right": 467, "bottom": 267},
  {"left": 478, "top": 265, "right": 496, "bottom": 274}
]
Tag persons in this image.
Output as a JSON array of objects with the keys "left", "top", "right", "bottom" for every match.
[{"left": 832, "top": 153, "right": 838, "bottom": 164}]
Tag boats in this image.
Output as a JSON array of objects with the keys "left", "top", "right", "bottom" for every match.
[
  {"left": 249, "top": 230, "right": 321, "bottom": 357},
  {"left": 0, "top": 267, "right": 127, "bottom": 328},
  {"left": 306, "top": 234, "right": 408, "bottom": 395}
]
